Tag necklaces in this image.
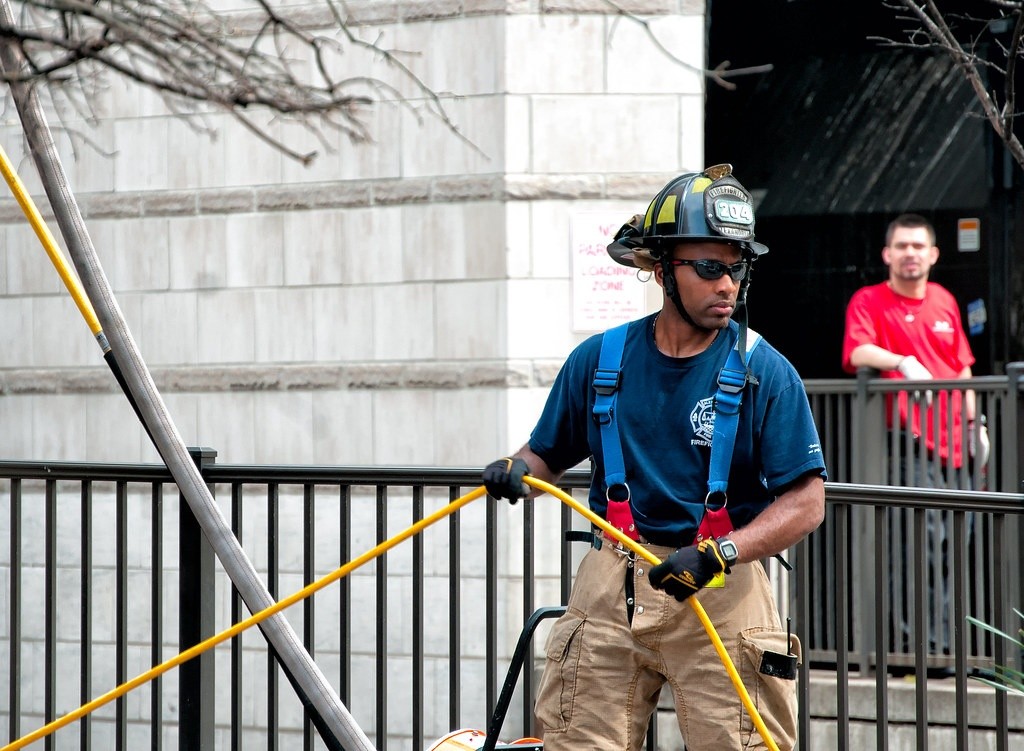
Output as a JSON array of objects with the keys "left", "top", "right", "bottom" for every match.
[{"left": 651, "top": 312, "right": 718, "bottom": 358}]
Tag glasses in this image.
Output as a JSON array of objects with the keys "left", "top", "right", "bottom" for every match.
[{"left": 669, "top": 254, "right": 748, "bottom": 280}]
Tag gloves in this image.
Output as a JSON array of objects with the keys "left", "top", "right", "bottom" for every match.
[
  {"left": 481, "top": 456, "right": 530, "bottom": 505},
  {"left": 648, "top": 541, "right": 725, "bottom": 602}
]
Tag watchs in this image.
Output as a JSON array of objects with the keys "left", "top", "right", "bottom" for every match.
[{"left": 715, "top": 538, "right": 738, "bottom": 568}]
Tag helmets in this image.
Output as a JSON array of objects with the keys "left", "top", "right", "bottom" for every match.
[{"left": 606, "top": 165, "right": 769, "bottom": 261}]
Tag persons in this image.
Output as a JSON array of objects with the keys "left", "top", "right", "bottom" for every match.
[
  {"left": 482, "top": 162, "right": 828, "bottom": 751},
  {"left": 841, "top": 214, "right": 991, "bottom": 678}
]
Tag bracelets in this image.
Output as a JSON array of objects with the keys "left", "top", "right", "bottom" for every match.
[
  {"left": 894, "top": 356, "right": 916, "bottom": 371},
  {"left": 966, "top": 414, "right": 987, "bottom": 425}
]
